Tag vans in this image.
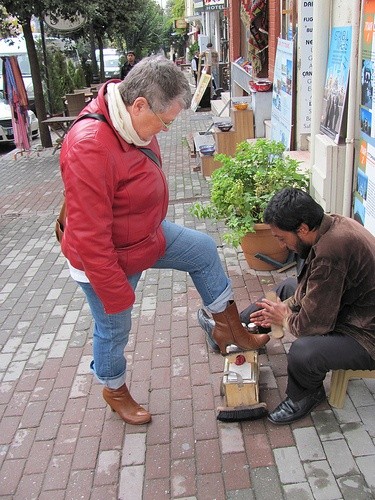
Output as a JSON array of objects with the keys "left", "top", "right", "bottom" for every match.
[
  {"left": 0, "top": 33, "right": 84, "bottom": 149},
  {"left": 94, "top": 49, "right": 122, "bottom": 79}
]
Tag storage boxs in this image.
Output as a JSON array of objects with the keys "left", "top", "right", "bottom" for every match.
[{"left": 223, "top": 350, "right": 259, "bottom": 407}]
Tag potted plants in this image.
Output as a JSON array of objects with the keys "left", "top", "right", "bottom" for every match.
[
  {"left": 44, "top": 45, "right": 87, "bottom": 131},
  {"left": 190, "top": 138, "right": 313, "bottom": 271}
]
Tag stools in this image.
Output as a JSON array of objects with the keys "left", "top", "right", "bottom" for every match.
[{"left": 327, "top": 369, "right": 375, "bottom": 409}]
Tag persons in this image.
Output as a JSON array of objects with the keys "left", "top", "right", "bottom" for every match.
[
  {"left": 61, "top": 56, "right": 271, "bottom": 424},
  {"left": 121, "top": 51, "right": 139, "bottom": 80},
  {"left": 196, "top": 187, "right": 375, "bottom": 425},
  {"left": 202, "top": 43, "right": 219, "bottom": 85},
  {"left": 192, "top": 52, "right": 200, "bottom": 87}
]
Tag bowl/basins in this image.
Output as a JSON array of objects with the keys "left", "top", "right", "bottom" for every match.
[
  {"left": 249, "top": 79, "right": 272, "bottom": 92},
  {"left": 233, "top": 103, "right": 248, "bottom": 110},
  {"left": 215, "top": 121, "right": 233, "bottom": 132},
  {"left": 199, "top": 144, "right": 216, "bottom": 156}
]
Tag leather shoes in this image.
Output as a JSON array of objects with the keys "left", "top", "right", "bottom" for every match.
[
  {"left": 266, "top": 382, "right": 326, "bottom": 426},
  {"left": 197, "top": 308, "right": 220, "bottom": 350}
]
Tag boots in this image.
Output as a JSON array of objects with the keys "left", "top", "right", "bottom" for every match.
[
  {"left": 103, "top": 384, "right": 151, "bottom": 425},
  {"left": 210, "top": 300, "right": 271, "bottom": 355}
]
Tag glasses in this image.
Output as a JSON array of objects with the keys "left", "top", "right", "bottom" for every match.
[{"left": 145, "top": 97, "right": 174, "bottom": 129}]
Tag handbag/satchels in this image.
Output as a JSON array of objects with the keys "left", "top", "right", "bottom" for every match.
[{"left": 56, "top": 193, "right": 69, "bottom": 242}]
